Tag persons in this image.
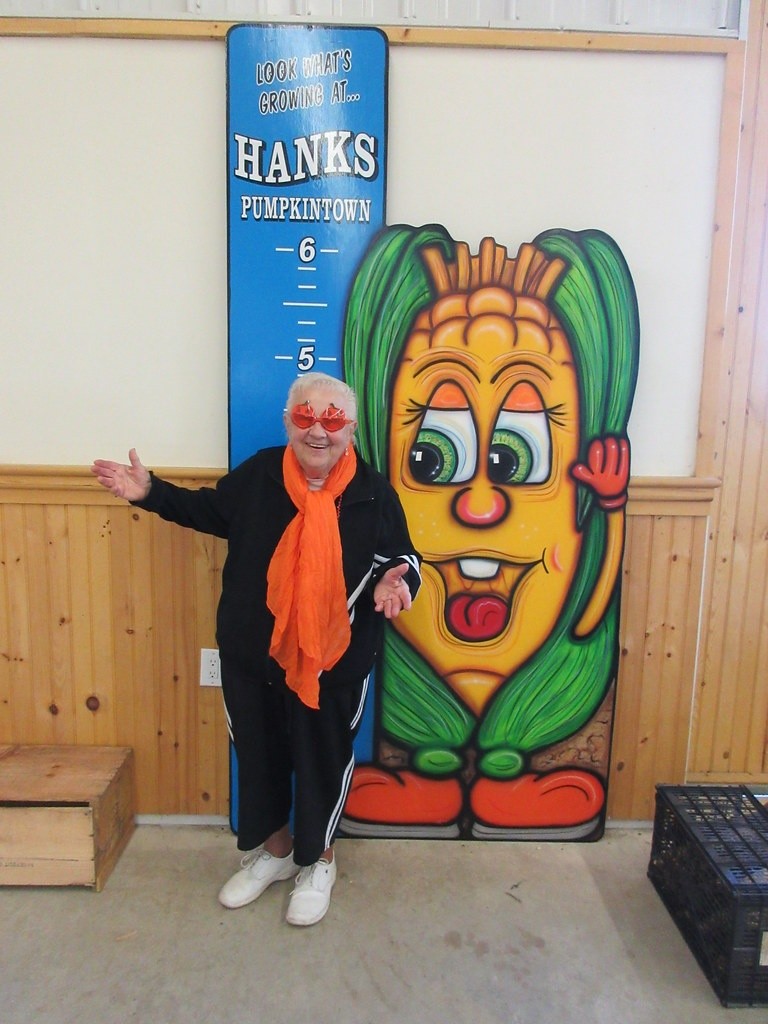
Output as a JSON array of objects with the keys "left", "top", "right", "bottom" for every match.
[{"left": 91, "top": 372, "right": 422, "bottom": 927}]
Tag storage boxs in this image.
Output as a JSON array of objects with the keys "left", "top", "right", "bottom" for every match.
[
  {"left": 643, "top": 780, "right": 768, "bottom": 1009},
  {"left": 1, "top": 743, "right": 145, "bottom": 893}
]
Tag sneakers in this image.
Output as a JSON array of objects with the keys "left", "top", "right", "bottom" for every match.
[
  {"left": 216, "top": 845, "right": 300, "bottom": 908},
  {"left": 285, "top": 847, "right": 336, "bottom": 922}
]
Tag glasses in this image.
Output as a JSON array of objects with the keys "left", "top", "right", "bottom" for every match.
[{"left": 281, "top": 402, "right": 358, "bottom": 433}]
